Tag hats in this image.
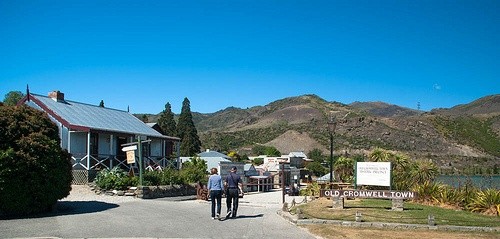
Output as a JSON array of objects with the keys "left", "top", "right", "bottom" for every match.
[{"left": 231, "top": 166, "right": 237, "bottom": 171}]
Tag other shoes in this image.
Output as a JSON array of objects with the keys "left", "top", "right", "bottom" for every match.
[
  {"left": 216, "top": 214, "right": 221, "bottom": 221},
  {"left": 226, "top": 211, "right": 231, "bottom": 218},
  {"left": 212, "top": 217, "right": 214, "bottom": 219}
]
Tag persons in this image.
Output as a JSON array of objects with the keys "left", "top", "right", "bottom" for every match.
[
  {"left": 206, "top": 168, "right": 225, "bottom": 220},
  {"left": 224, "top": 166, "right": 245, "bottom": 220}
]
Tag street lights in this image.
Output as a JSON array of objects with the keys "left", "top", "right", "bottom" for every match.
[{"left": 325, "top": 117, "right": 337, "bottom": 189}]
